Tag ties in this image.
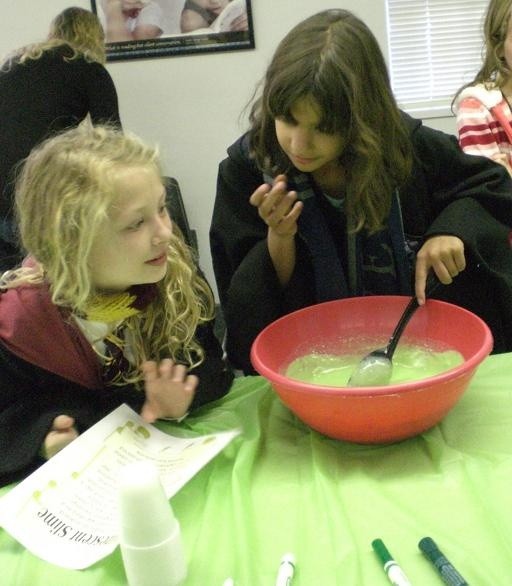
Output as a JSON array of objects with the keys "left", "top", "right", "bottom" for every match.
[{"left": 102, "top": 330, "right": 130, "bottom": 381}]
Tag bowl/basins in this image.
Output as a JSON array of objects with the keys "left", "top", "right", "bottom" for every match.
[{"left": 246, "top": 294, "right": 492, "bottom": 448}]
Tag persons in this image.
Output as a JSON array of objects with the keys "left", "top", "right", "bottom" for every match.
[
  {"left": 451, "top": 0, "right": 512, "bottom": 180},
  {"left": 0, "top": 114, "right": 235, "bottom": 476},
  {"left": 95, "top": 0, "right": 164, "bottom": 41},
  {"left": 209, "top": 8, "right": 512, "bottom": 376},
  {"left": 0, "top": 7, "right": 125, "bottom": 274},
  {"left": 180, "top": 0, "right": 250, "bottom": 33}
]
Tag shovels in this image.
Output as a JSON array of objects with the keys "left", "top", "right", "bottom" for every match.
[{"left": 346, "top": 272, "right": 442, "bottom": 386}]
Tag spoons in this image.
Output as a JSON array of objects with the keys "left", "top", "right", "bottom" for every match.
[{"left": 349, "top": 263, "right": 451, "bottom": 389}]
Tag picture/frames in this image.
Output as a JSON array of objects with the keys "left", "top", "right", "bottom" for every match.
[{"left": 91, "top": 0, "right": 255, "bottom": 61}]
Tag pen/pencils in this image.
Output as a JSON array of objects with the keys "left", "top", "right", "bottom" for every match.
[{"left": 275, "top": 552, "right": 297, "bottom": 585}]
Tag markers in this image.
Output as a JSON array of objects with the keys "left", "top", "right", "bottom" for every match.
[
  {"left": 415, "top": 535, "right": 472, "bottom": 586},
  {"left": 367, "top": 537, "right": 411, "bottom": 586}
]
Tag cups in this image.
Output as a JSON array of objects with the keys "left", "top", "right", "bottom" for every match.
[{"left": 109, "top": 466, "right": 189, "bottom": 586}]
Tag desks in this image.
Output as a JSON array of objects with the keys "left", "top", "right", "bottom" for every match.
[{"left": 0, "top": 349, "right": 512, "bottom": 586}]
